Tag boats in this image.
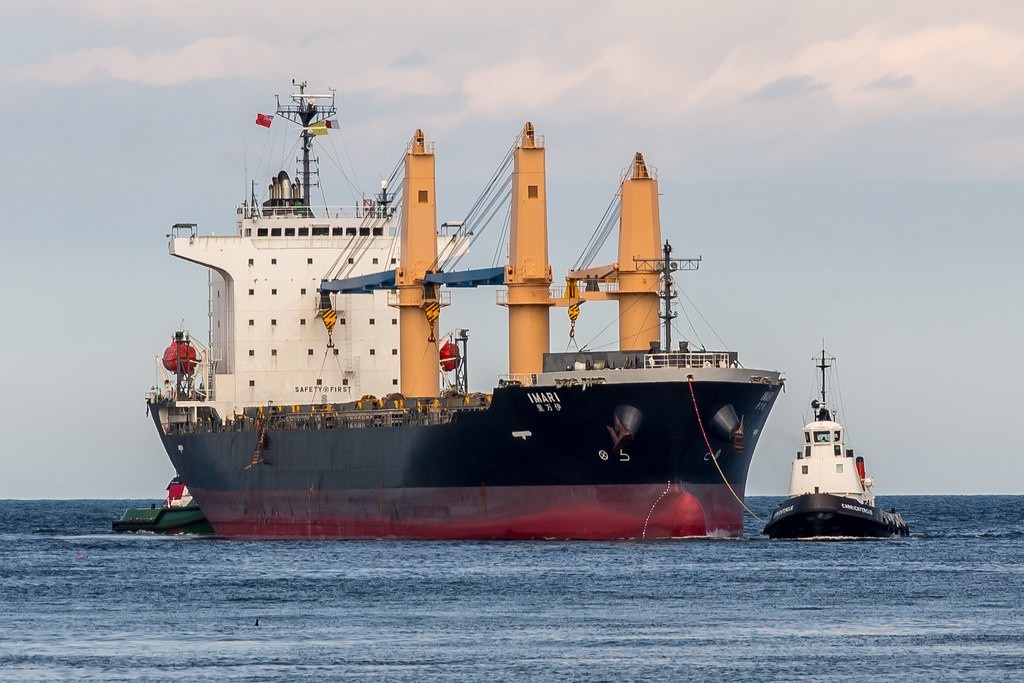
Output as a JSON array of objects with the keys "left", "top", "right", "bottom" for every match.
[
  {"left": 111, "top": 476, "right": 215, "bottom": 534},
  {"left": 763, "top": 336, "right": 910, "bottom": 538},
  {"left": 142, "top": 72, "right": 786, "bottom": 540}
]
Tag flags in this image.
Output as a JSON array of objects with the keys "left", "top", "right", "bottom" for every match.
[
  {"left": 309, "top": 121, "right": 329, "bottom": 135},
  {"left": 255, "top": 113, "right": 274, "bottom": 128},
  {"left": 326, "top": 120, "right": 340, "bottom": 129}
]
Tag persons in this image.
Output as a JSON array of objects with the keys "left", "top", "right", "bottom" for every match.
[
  {"left": 731, "top": 360, "right": 737, "bottom": 368},
  {"left": 648, "top": 355, "right": 660, "bottom": 368}
]
